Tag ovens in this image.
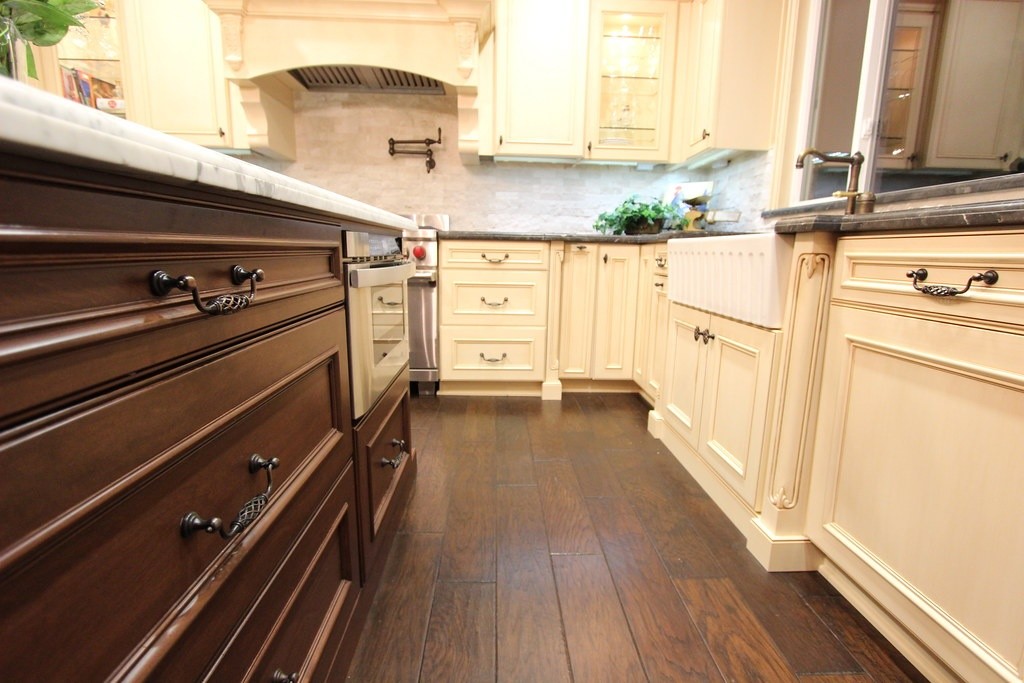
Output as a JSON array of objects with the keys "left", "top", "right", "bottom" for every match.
[{"left": 343, "top": 230, "right": 417, "bottom": 421}]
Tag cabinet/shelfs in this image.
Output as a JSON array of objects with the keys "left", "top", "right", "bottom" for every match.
[
  {"left": 875, "top": 0, "right": 1024, "bottom": 174},
  {"left": 439, "top": 240, "right": 1024, "bottom": 683},
  {"left": 117, "top": 0, "right": 249, "bottom": 152},
  {"left": 0, "top": 156, "right": 415, "bottom": 683},
  {"left": 480, "top": 0, "right": 787, "bottom": 171}
]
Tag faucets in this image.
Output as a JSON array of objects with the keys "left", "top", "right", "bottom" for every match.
[{"left": 796, "top": 147, "right": 865, "bottom": 215}]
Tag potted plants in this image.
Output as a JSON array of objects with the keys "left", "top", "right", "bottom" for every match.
[{"left": 593, "top": 195, "right": 689, "bottom": 235}]
